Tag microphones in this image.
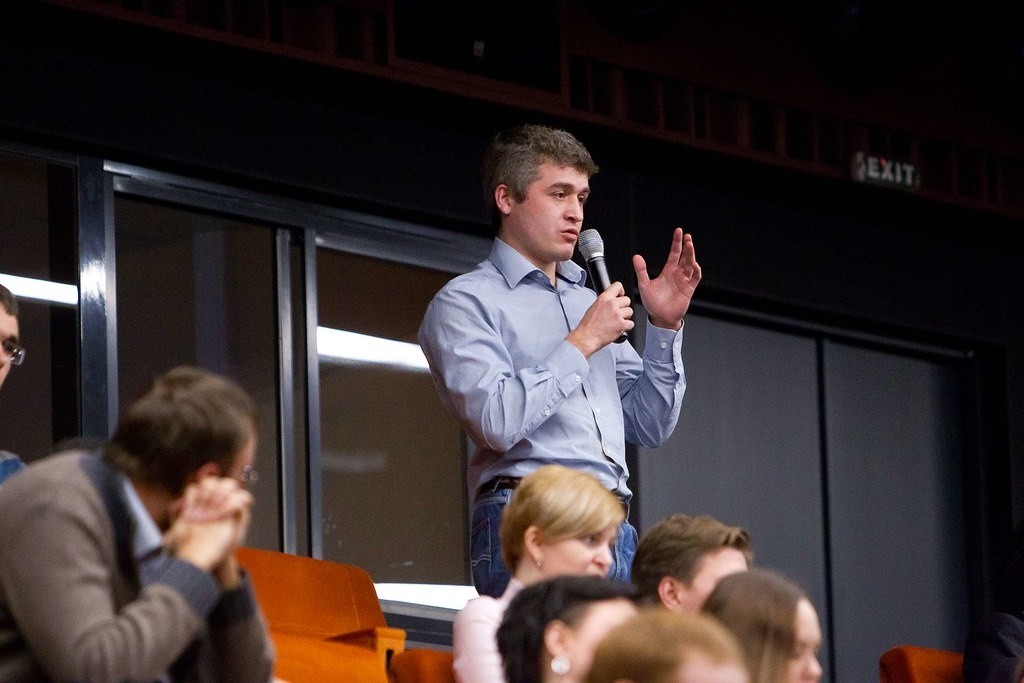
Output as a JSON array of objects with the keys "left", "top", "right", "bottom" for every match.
[{"left": 578, "top": 229, "right": 628, "bottom": 344}]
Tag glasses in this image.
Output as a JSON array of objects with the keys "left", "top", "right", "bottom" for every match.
[{"left": 0, "top": 338, "right": 26, "bottom": 366}]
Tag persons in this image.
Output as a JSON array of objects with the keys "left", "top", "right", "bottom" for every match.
[
  {"left": 497, "top": 576, "right": 644, "bottom": 683},
  {"left": 963, "top": 612, "right": 1024, "bottom": 683},
  {"left": 629, "top": 514, "right": 752, "bottom": 616},
  {"left": 705, "top": 568, "right": 824, "bottom": 683},
  {"left": 0, "top": 284, "right": 26, "bottom": 483},
  {"left": 417, "top": 124, "right": 703, "bottom": 593},
  {"left": 0, "top": 366, "right": 275, "bottom": 683},
  {"left": 450, "top": 464, "right": 624, "bottom": 683},
  {"left": 583, "top": 605, "right": 756, "bottom": 683}
]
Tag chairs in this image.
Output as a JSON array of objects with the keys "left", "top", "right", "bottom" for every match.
[{"left": 239, "top": 553, "right": 965, "bottom": 683}]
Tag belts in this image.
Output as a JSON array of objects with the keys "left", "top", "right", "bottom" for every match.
[{"left": 490, "top": 475, "right": 628, "bottom": 524}]
